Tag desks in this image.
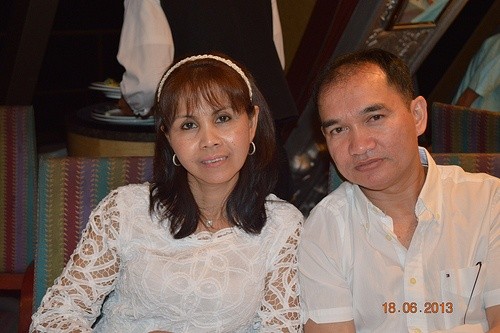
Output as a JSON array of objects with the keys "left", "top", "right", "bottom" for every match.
[{"left": 66, "top": 100, "right": 158, "bottom": 159}]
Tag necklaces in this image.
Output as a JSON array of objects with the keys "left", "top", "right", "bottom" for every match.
[{"left": 206, "top": 219, "right": 213, "bottom": 227}]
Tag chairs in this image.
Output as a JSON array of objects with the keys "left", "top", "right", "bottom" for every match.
[
  {"left": 328, "top": 101, "right": 500, "bottom": 196},
  {"left": 0, "top": 102, "right": 161, "bottom": 333}
]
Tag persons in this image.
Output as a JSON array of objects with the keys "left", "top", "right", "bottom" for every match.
[
  {"left": 453, "top": 34, "right": 500, "bottom": 111},
  {"left": 29, "top": 53, "right": 306, "bottom": 333},
  {"left": 116, "top": 0, "right": 297, "bottom": 196},
  {"left": 297, "top": 48, "right": 500, "bottom": 333}
]
